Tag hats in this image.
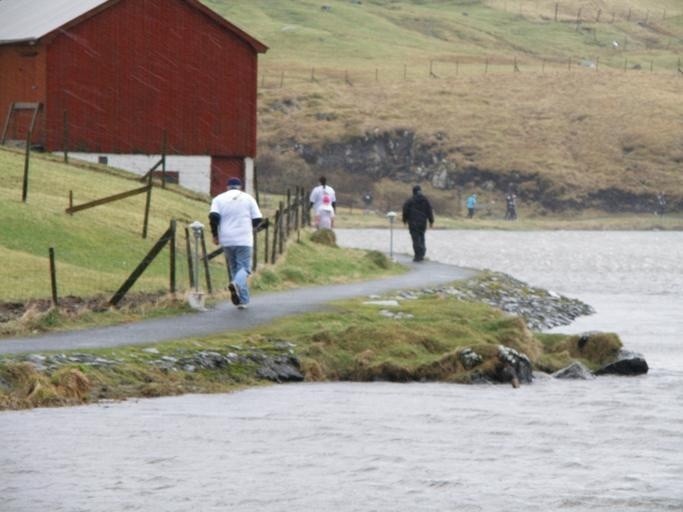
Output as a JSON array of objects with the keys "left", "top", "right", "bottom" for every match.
[{"left": 226, "top": 178, "right": 241, "bottom": 186}]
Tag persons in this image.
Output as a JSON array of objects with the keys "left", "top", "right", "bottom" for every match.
[
  {"left": 653, "top": 190, "right": 666, "bottom": 219},
  {"left": 505, "top": 192, "right": 517, "bottom": 220},
  {"left": 401, "top": 185, "right": 433, "bottom": 262},
  {"left": 208, "top": 176, "right": 262, "bottom": 309},
  {"left": 464, "top": 191, "right": 477, "bottom": 218},
  {"left": 314, "top": 195, "right": 335, "bottom": 230},
  {"left": 306, "top": 174, "right": 337, "bottom": 231}
]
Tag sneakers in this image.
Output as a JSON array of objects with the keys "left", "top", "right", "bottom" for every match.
[{"left": 228, "top": 282, "right": 247, "bottom": 309}]
